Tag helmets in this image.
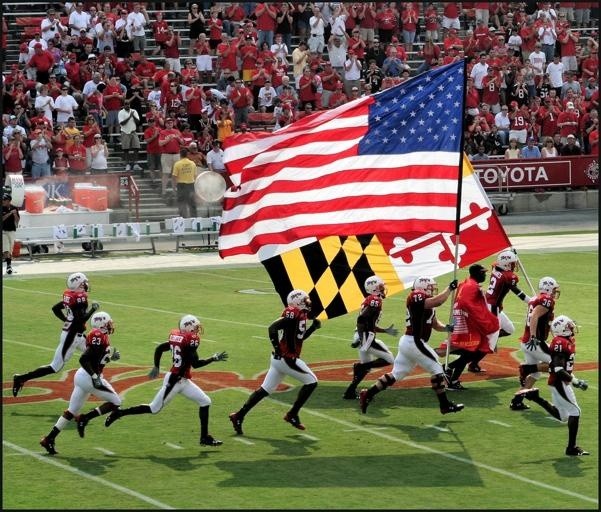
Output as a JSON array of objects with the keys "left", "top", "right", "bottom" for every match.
[
  {"left": 67, "top": 273, "right": 89, "bottom": 291},
  {"left": 364, "top": 276, "right": 387, "bottom": 298},
  {"left": 551, "top": 316, "right": 575, "bottom": 337},
  {"left": 179, "top": 315, "right": 203, "bottom": 333},
  {"left": 539, "top": 277, "right": 560, "bottom": 299},
  {"left": 496, "top": 251, "right": 518, "bottom": 271},
  {"left": 287, "top": 290, "right": 311, "bottom": 311},
  {"left": 413, "top": 276, "right": 438, "bottom": 295},
  {"left": 91, "top": 311, "right": 114, "bottom": 334}
]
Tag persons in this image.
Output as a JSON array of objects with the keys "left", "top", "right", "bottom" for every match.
[
  {"left": 435, "top": 264, "right": 500, "bottom": 391},
  {"left": 2, "top": 1, "right": 221, "bottom": 274},
  {"left": 515, "top": 314, "right": 592, "bottom": 457},
  {"left": 469, "top": 251, "right": 534, "bottom": 375},
  {"left": 344, "top": 275, "right": 399, "bottom": 400},
  {"left": 509, "top": 277, "right": 562, "bottom": 410},
  {"left": 40, "top": 310, "right": 123, "bottom": 454},
  {"left": 104, "top": 314, "right": 229, "bottom": 448},
  {"left": 12, "top": 271, "right": 101, "bottom": 397},
  {"left": 230, "top": 290, "right": 323, "bottom": 436},
  {"left": 357, "top": 277, "right": 466, "bottom": 414},
  {"left": 224, "top": 1, "right": 599, "bottom": 190}
]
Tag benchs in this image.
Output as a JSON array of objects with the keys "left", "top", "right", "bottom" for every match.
[
  {"left": 165, "top": 216, "right": 219, "bottom": 253},
  {"left": 15, "top": 222, "right": 170, "bottom": 260}
]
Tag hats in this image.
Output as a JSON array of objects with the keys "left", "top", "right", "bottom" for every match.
[
  {"left": 488, "top": 27, "right": 496, "bottom": 31},
  {"left": 502, "top": 97, "right": 575, "bottom": 109},
  {"left": 469, "top": 264, "right": 488, "bottom": 272}
]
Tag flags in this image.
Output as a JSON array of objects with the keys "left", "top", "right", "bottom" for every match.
[
  {"left": 260, "top": 153, "right": 510, "bottom": 322},
  {"left": 217, "top": 58, "right": 466, "bottom": 264}
]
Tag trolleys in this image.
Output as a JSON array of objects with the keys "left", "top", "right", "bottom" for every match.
[
  {"left": 15, "top": 208, "right": 113, "bottom": 253},
  {"left": 474, "top": 165, "right": 516, "bottom": 216}
]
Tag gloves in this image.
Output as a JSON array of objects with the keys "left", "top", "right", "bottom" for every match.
[
  {"left": 313, "top": 318, "right": 320, "bottom": 329},
  {"left": 385, "top": 324, "right": 398, "bottom": 335},
  {"left": 449, "top": 280, "right": 457, "bottom": 291},
  {"left": 446, "top": 324, "right": 453, "bottom": 332},
  {"left": 111, "top": 348, "right": 119, "bottom": 360},
  {"left": 573, "top": 380, "right": 588, "bottom": 390},
  {"left": 213, "top": 352, "right": 228, "bottom": 361},
  {"left": 150, "top": 367, "right": 158, "bottom": 379},
  {"left": 274, "top": 349, "right": 282, "bottom": 359},
  {"left": 92, "top": 302, "right": 99, "bottom": 310},
  {"left": 94, "top": 378, "right": 102, "bottom": 387}
]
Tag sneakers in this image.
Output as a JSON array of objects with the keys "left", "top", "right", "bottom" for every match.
[
  {"left": 442, "top": 364, "right": 486, "bottom": 390},
  {"left": 40, "top": 408, "right": 119, "bottom": 453},
  {"left": 229, "top": 413, "right": 243, "bottom": 434},
  {"left": 510, "top": 388, "right": 538, "bottom": 410},
  {"left": 342, "top": 363, "right": 371, "bottom": 413},
  {"left": 125, "top": 164, "right": 143, "bottom": 171},
  {"left": 13, "top": 375, "right": 23, "bottom": 396},
  {"left": 200, "top": 435, "right": 223, "bottom": 446},
  {"left": 284, "top": 413, "right": 305, "bottom": 430},
  {"left": 441, "top": 402, "right": 463, "bottom": 414},
  {"left": 566, "top": 446, "right": 589, "bottom": 455}
]
